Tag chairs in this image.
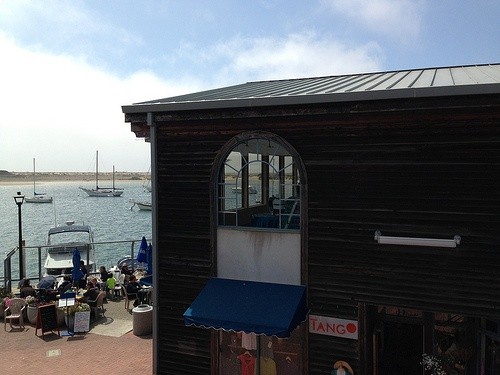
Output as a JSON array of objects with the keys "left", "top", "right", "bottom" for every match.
[
  {"left": 71, "top": 271, "right": 138, "bottom": 320},
  {"left": 4, "top": 298, "right": 25, "bottom": 332}
]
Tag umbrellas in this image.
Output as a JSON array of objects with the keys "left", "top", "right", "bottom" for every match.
[
  {"left": 70, "top": 249, "right": 84, "bottom": 290},
  {"left": 36, "top": 275, "right": 56, "bottom": 289},
  {"left": 137, "top": 236, "right": 152, "bottom": 275}
]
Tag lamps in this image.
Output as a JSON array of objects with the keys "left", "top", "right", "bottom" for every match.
[{"left": 375, "top": 231, "right": 460, "bottom": 247}]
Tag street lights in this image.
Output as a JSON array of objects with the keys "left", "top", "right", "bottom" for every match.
[{"left": 13, "top": 191, "right": 26, "bottom": 282}]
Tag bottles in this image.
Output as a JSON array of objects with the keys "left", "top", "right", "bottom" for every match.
[{"left": 131, "top": 304, "right": 153, "bottom": 336}]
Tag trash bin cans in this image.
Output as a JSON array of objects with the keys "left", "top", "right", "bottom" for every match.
[{"left": 132, "top": 304, "right": 154, "bottom": 336}]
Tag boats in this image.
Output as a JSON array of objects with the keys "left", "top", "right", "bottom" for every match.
[
  {"left": 42, "top": 201, "right": 98, "bottom": 288},
  {"left": 231, "top": 184, "right": 258, "bottom": 194},
  {"left": 130, "top": 198, "right": 152, "bottom": 211}
]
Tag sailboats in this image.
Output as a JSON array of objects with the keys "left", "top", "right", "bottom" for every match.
[
  {"left": 140, "top": 165, "right": 152, "bottom": 192},
  {"left": 78, "top": 150, "right": 125, "bottom": 198},
  {"left": 24, "top": 156, "right": 54, "bottom": 203}
]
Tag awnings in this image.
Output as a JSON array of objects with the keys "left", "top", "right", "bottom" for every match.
[{"left": 182, "top": 277, "right": 310, "bottom": 340}]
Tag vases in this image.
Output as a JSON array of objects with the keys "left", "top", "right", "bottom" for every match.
[
  {"left": 56, "top": 309, "right": 64, "bottom": 327},
  {"left": 27, "top": 305, "right": 54, "bottom": 324},
  {"left": 0, "top": 299, "right": 6, "bottom": 318},
  {"left": 64, "top": 313, "right": 74, "bottom": 329}
]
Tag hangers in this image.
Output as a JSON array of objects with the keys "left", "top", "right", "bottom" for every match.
[
  {"left": 237, "top": 351, "right": 252, "bottom": 359},
  {"left": 285, "top": 355, "right": 291, "bottom": 361}
]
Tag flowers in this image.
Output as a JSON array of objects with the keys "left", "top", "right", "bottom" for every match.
[
  {"left": 64, "top": 302, "right": 90, "bottom": 316},
  {"left": 0, "top": 286, "right": 16, "bottom": 306},
  {"left": 26, "top": 295, "right": 54, "bottom": 307},
  {"left": 53, "top": 301, "right": 68, "bottom": 310}
]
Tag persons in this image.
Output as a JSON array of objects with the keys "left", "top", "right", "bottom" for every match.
[
  {"left": 100, "top": 266, "right": 109, "bottom": 282},
  {"left": 80, "top": 261, "right": 87, "bottom": 288},
  {"left": 125, "top": 275, "right": 144, "bottom": 307},
  {"left": 105, "top": 272, "right": 116, "bottom": 292},
  {"left": 121, "top": 263, "right": 133, "bottom": 276},
  {"left": 20, "top": 275, "right": 101, "bottom": 307}
]
